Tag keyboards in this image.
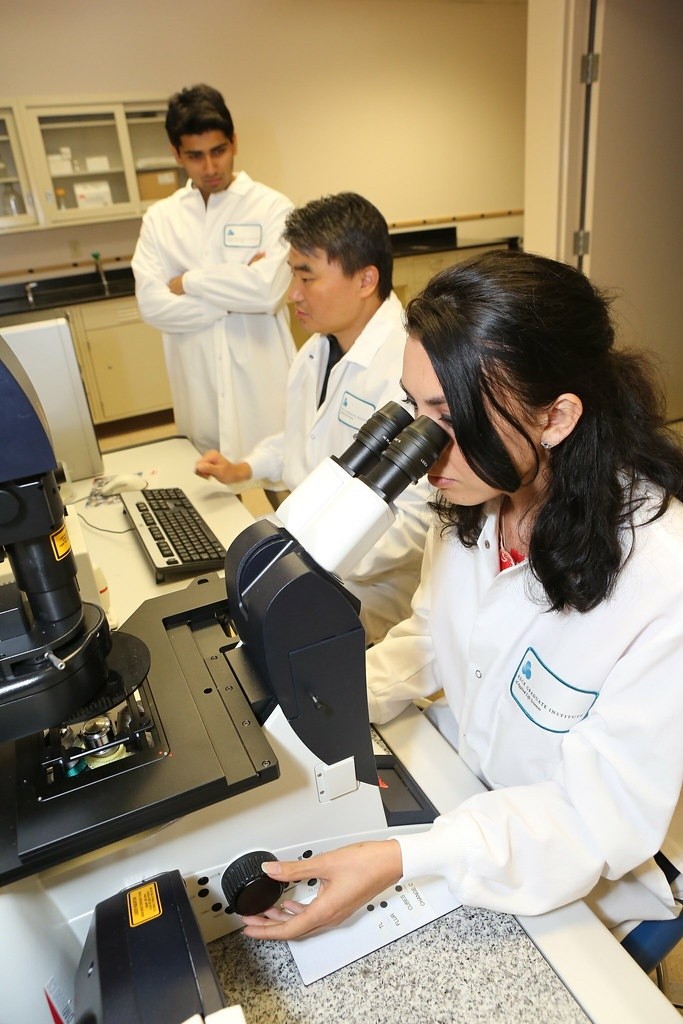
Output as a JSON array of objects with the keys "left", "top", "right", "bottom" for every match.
[{"left": 120, "top": 487, "right": 228, "bottom": 584}]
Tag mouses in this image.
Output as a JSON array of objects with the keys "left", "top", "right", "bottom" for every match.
[{"left": 99, "top": 474, "right": 148, "bottom": 498}]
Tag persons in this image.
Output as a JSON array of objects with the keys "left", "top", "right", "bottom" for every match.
[
  {"left": 241, "top": 250, "right": 683, "bottom": 974},
  {"left": 131, "top": 84, "right": 297, "bottom": 504},
  {"left": 194, "top": 190, "right": 437, "bottom": 647}
]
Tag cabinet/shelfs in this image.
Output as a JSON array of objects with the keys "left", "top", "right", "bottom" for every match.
[
  {"left": 0, "top": 87, "right": 191, "bottom": 234},
  {"left": 282, "top": 245, "right": 509, "bottom": 354},
  {"left": 0, "top": 292, "right": 179, "bottom": 426}
]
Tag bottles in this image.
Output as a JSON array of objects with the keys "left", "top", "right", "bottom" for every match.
[
  {"left": 2, "top": 183, "right": 23, "bottom": 216},
  {"left": 55, "top": 188, "right": 68, "bottom": 210}
]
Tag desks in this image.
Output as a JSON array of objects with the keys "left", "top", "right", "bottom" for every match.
[{"left": 0, "top": 437, "right": 682, "bottom": 1024}]
